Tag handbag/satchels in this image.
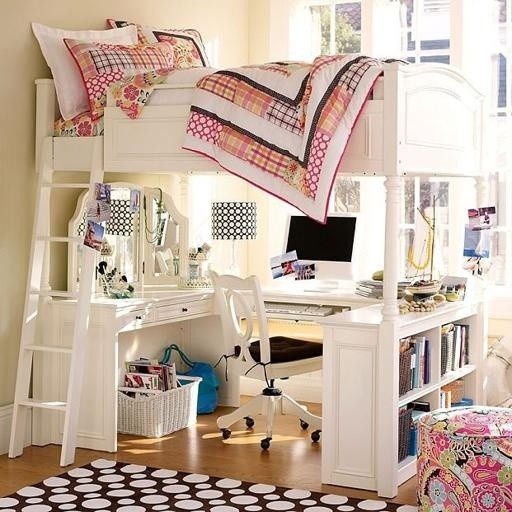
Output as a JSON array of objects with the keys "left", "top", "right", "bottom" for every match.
[{"left": 161, "top": 344, "right": 218, "bottom": 414}]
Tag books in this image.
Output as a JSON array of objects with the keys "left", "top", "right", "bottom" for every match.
[
  {"left": 408, "top": 323, "right": 469, "bottom": 429},
  {"left": 355, "top": 280, "right": 411, "bottom": 298},
  {"left": 124, "top": 358, "right": 177, "bottom": 398}
]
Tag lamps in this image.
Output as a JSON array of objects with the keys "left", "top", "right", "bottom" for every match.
[
  {"left": 105, "top": 199, "right": 139, "bottom": 275},
  {"left": 211, "top": 201, "right": 257, "bottom": 264}
]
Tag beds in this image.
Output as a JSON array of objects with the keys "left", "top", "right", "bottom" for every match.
[{"left": 34, "top": 54, "right": 497, "bottom": 178}]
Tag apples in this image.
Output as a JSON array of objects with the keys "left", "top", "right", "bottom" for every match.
[
  {"left": 372, "top": 270, "right": 383, "bottom": 281},
  {"left": 446, "top": 292, "right": 457, "bottom": 302},
  {"left": 433, "top": 294, "right": 445, "bottom": 304}
]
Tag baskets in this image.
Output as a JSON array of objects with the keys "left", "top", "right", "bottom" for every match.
[
  {"left": 118, "top": 373, "right": 203, "bottom": 439},
  {"left": 441, "top": 380, "right": 464, "bottom": 403},
  {"left": 398, "top": 408, "right": 413, "bottom": 460},
  {"left": 399, "top": 347, "right": 414, "bottom": 394}
]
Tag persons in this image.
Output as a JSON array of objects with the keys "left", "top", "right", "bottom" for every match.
[
  {"left": 84, "top": 226, "right": 102, "bottom": 251},
  {"left": 483, "top": 210, "right": 490, "bottom": 225},
  {"left": 96, "top": 183, "right": 102, "bottom": 200},
  {"left": 104, "top": 185, "right": 111, "bottom": 201},
  {"left": 281, "top": 261, "right": 312, "bottom": 279}
]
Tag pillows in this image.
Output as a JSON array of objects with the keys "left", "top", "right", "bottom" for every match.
[
  {"left": 107, "top": 18, "right": 212, "bottom": 68},
  {"left": 32, "top": 21, "right": 138, "bottom": 121},
  {"left": 62, "top": 37, "right": 174, "bottom": 121}
]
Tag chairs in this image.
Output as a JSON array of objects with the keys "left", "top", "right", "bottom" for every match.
[
  {"left": 205, "top": 265, "right": 323, "bottom": 450},
  {"left": 154, "top": 248, "right": 174, "bottom": 276}
]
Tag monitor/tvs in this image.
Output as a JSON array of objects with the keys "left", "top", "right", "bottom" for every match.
[{"left": 284, "top": 215, "right": 358, "bottom": 289}]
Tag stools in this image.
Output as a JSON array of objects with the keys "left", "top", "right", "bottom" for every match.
[{"left": 416, "top": 404, "right": 512, "bottom": 511}]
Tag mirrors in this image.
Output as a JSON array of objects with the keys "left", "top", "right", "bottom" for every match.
[
  {"left": 67, "top": 181, "right": 144, "bottom": 292},
  {"left": 142, "top": 185, "right": 190, "bottom": 285}
]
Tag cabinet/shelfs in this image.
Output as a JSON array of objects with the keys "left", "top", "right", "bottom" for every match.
[{"left": 48, "top": 279, "right": 489, "bottom": 500}]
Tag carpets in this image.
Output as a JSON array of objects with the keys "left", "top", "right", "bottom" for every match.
[{"left": 1, "top": 457, "right": 417, "bottom": 512}]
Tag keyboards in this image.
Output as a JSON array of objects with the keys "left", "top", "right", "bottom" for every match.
[{"left": 253, "top": 303, "right": 333, "bottom": 317}]
